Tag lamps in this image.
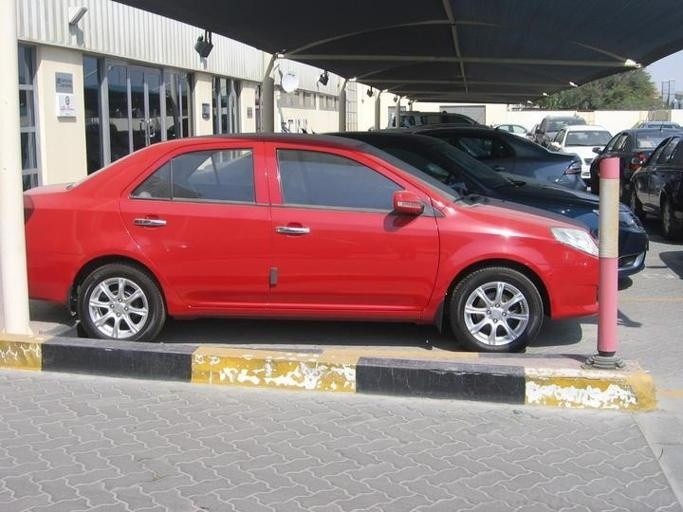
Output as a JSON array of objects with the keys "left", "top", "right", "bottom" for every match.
[{"left": 194, "top": 28, "right": 416, "bottom": 106}]
